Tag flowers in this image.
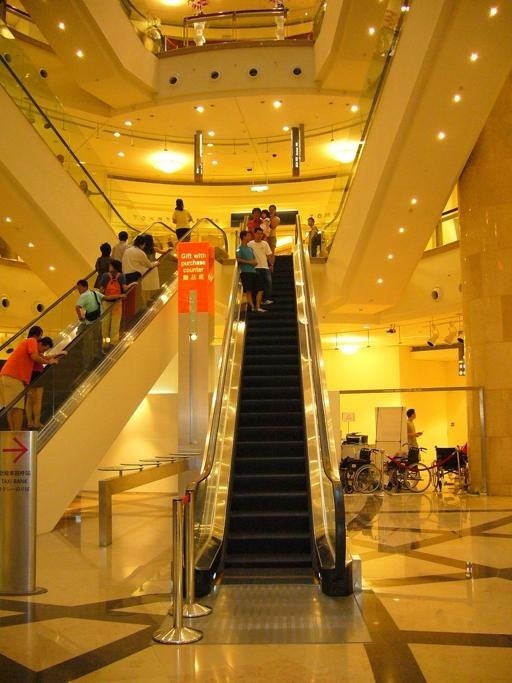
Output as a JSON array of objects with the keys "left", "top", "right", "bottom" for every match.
[
  {"left": 268, "top": 0, "right": 289, "bottom": 7},
  {"left": 187, "top": 0, "right": 210, "bottom": 15}
]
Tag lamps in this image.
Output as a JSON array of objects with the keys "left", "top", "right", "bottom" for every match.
[{"left": 426, "top": 313, "right": 465, "bottom": 347}]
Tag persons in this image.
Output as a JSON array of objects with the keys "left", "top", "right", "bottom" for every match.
[
  {"left": 0, "top": 326, "right": 60, "bottom": 431},
  {"left": 405, "top": 409, "right": 422, "bottom": 480},
  {"left": 172, "top": 199, "right": 193, "bottom": 242},
  {"left": 308, "top": 217, "right": 318, "bottom": 256},
  {"left": 76, "top": 231, "right": 173, "bottom": 369},
  {"left": 235, "top": 205, "right": 280, "bottom": 312},
  {"left": 25, "top": 337, "right": 68, "bottom": 427}
]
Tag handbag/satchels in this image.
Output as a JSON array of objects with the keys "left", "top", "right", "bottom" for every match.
[
  {"left": 311, "top": 234, "right": 321, "bottom": 245},
  {"left": 85, "top": 307, "right": 100, "bottom": 321}
]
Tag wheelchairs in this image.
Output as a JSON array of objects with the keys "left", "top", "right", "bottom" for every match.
[
  {"left": 434, "top": 444, "right": 469, "bottom": 493},
  {"left": 388, "top": 442, "right": 432, "bottom": 494},
  {"left": 434, "top": 494, "right": 470, "bottom": 538},
  {"left": 340, "top": 447, "right": 382, "bottom": 493},
  {"left": 393, "top": 494, "right": 432, "bottom": 521}
]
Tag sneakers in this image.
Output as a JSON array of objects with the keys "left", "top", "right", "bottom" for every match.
[
  {"left": 261, "top": 299, "right": 274, "bottom": 304},
  {"left": 256, "top": 308, "right": 267, "bottom": 312}
]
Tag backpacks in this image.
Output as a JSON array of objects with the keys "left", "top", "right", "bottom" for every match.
[{"left": 104, "top": 271, "right": 121, "bottom": 295}]
